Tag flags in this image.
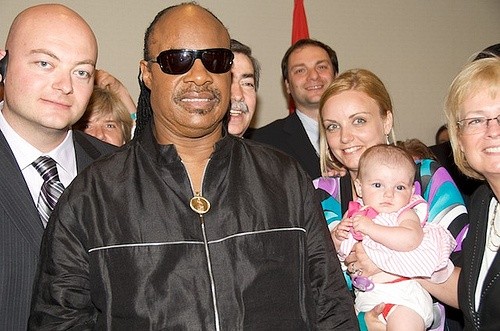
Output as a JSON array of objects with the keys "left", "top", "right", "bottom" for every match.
[{"left": 289, "top": 0, "right": 309, "bottom": 114}]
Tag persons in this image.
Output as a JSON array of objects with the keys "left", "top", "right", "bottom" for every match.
[
  {"left": 227, "top": 38, "right": 261, "bottom": 140},
  {"left": 70, "top": 69, "right": 137, "bottom": 148},
  {"left": 331, "top": 144, "right": 457, "bottom": 331},
  {"left": 435, "top": 123, "right": 451, "bottom": 145},
  {"left": 311, "top": 69, "right": 469, "bottom": 331},
  {"left": 413, "top": 51, "right": 499, "bottom": 331},
  {"left": 28, "top": 3, "right": 359, "bottom": 331},
  {"left": 246, "top": 38, "right": 339, "bottom": 180},
  {"left": 1, "top": 4, "right": 122, "bottom": 330}
]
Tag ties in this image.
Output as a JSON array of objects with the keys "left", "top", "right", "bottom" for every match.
[{"left": 31, "top": 155, "right": 65, "bottom": 221}]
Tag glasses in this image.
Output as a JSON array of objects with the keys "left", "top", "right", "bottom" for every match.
[
  {"left": 150, "top": 48, "right": 233, "bottom": 74},
  {"left": 457, "top": 114, "right": 500, "bottom": 135}
]
